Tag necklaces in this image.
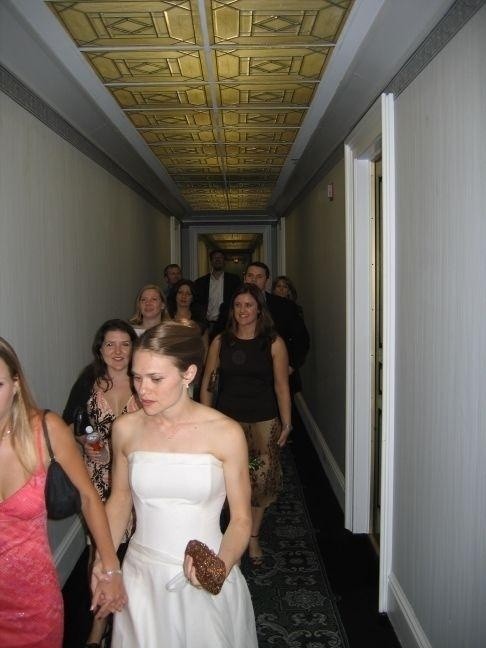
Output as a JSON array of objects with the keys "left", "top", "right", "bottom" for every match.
[{"left": 0, "top": 422, "right": 11, "bottom": 443}]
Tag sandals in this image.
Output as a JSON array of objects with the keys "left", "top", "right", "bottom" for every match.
[{"left": 247, "top": 535, "right": 264, "bottom": 566}]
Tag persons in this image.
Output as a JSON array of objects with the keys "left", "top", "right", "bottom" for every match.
[
  {"left": 200, "top": 283, "right": 292, "bottom": 566},
  {"left": 0, "top": 335, "right": 130, "bottom": 648},
  {"left": 129, "top": 249, "right": 311, "bottom": 391},
  {"left": 61, "top": 318, "right": 142, "bottom": 647},
  {"left": 87, "top": 316, "right": 261, "bottom": 648}
]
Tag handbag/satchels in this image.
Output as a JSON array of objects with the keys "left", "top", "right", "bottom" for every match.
[
  {"left": 186, "top": 542, "right": 225, "bottom": 595},
  {"left": 74, "top": 407, "right": 90, "bottom": 435},
  {"left": 207, "top": 371, "right": 220, "bottom": 394},
  {"left": 42, "top": 461, "right": 81, "bottom": 522}
]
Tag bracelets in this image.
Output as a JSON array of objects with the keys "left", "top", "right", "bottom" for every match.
[{"left": 102, "top": 568, "right": 122, "bottom": 578}]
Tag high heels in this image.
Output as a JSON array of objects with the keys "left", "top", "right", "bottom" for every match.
[{"left": 86, "top": 619, "right": 110, "bottom": 648}]
